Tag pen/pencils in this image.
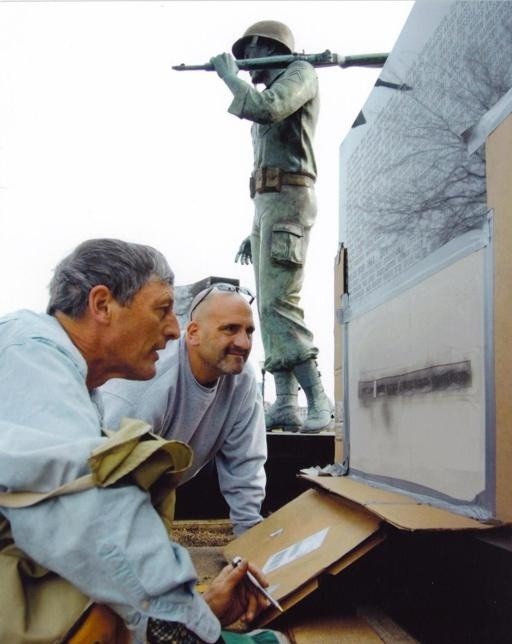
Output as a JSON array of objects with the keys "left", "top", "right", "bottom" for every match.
[{"left": 231, "top": 556, "right": 286, "bottom": 616}]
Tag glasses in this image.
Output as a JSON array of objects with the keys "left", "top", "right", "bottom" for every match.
[{"left": 190, "top": 282, "right": 254, "bottom": 322}]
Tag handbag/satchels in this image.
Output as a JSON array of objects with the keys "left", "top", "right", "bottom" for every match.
[{"left": 0, "top": 415, "right": 194, "bottom": 644}]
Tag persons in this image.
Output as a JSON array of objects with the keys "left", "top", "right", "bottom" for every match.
[
  {"left": 0, "top": 237, "right": 269, "bottom": 644},
  {"left": 209, "top": 19, "right": 335, "bottom": 433},
  {"left": 99, "top": 281, "right": 271, "bottom": 536}
]
